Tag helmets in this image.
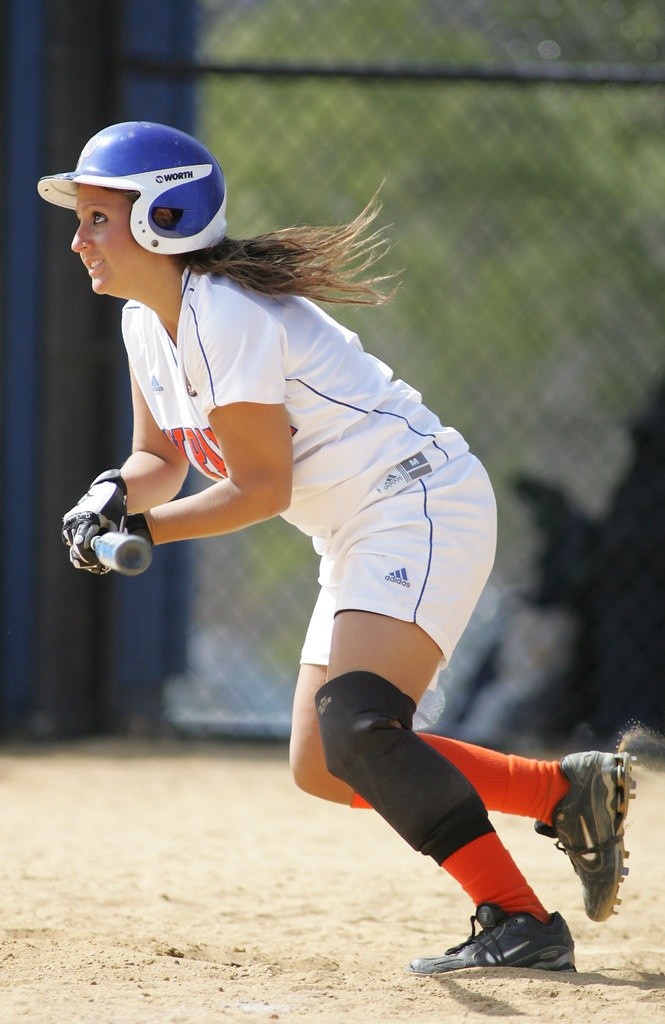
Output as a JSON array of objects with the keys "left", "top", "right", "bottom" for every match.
[{"left": 37, "top": 120, "right": 226, "bottom": 254}]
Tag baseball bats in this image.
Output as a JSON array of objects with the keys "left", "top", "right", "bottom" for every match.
[{"left": 86, "top": 528, "right": 154, "bottom": 578}]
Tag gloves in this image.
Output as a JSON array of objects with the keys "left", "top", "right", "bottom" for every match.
[{"left": 60, "top": 469, "right": 154, "bottom": 573}]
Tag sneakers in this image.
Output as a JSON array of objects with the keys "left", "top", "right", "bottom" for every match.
[
  {"left": 535, "top": 750, "right": 637, "bottom": 922},
  {"left": 407, "top": 901, "right": 575, "bottom": 974}
]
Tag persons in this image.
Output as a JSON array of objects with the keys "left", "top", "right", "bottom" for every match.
[{"left": 37, "top": 122, "right": 638, "bottom": 971}]
